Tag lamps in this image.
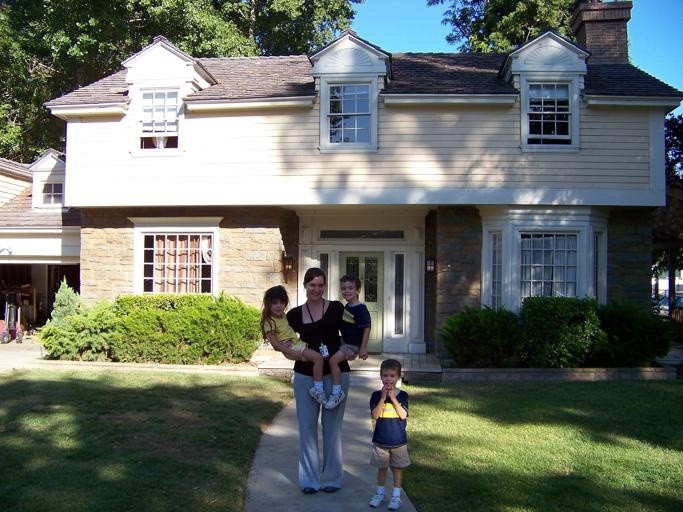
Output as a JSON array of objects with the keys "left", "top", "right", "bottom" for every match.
[
  {"left": 282, "top": 254, "right": 297, "bottom": 273},
  {"left": 424, "top": 256, "right": 435, "bottom": 273}
]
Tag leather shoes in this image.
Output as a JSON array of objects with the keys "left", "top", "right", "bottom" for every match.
[
  {"left": 324, "top": 487, "right": 336, "bottom": 493},
  {"left": 304, "top": 488, "right": 314, "bottom": 494}
]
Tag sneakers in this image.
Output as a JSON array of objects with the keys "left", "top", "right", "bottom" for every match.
[
  {"left": 325, "top": 390, "right": 345, "bottom": 410},
  {"left": 388, "top": 495, "right": 401, "bottom": 510},
  {"left": 369, "top": 490, "right": 385, "bottom": 508},
  {"left": 309, "top": 386, "right": 327, "bottom": 405}
]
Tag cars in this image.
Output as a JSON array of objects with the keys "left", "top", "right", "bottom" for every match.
[
  {"left": 653, "top": 283, "right": 682, "bottom": 325},
  {"left": 651, "top": 297, "right": 659, "bottom": 313}
]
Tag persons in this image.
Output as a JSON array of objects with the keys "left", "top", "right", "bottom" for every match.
[
  {"left": 259, "top": 284, "right": 327, "bottom": 408},
  {"left": 324, "top": 274, "right": 371, "bottom": 411},
  {"left": 367, "top": 357, "right": 411, "bottom": 510},
  {"left": 272, "top": 267, "right": 357, "bottom": 495}
]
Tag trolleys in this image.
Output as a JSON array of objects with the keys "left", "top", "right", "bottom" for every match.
[{"left": 1, "top": 288, "right": 24, "bottom": 345}]
